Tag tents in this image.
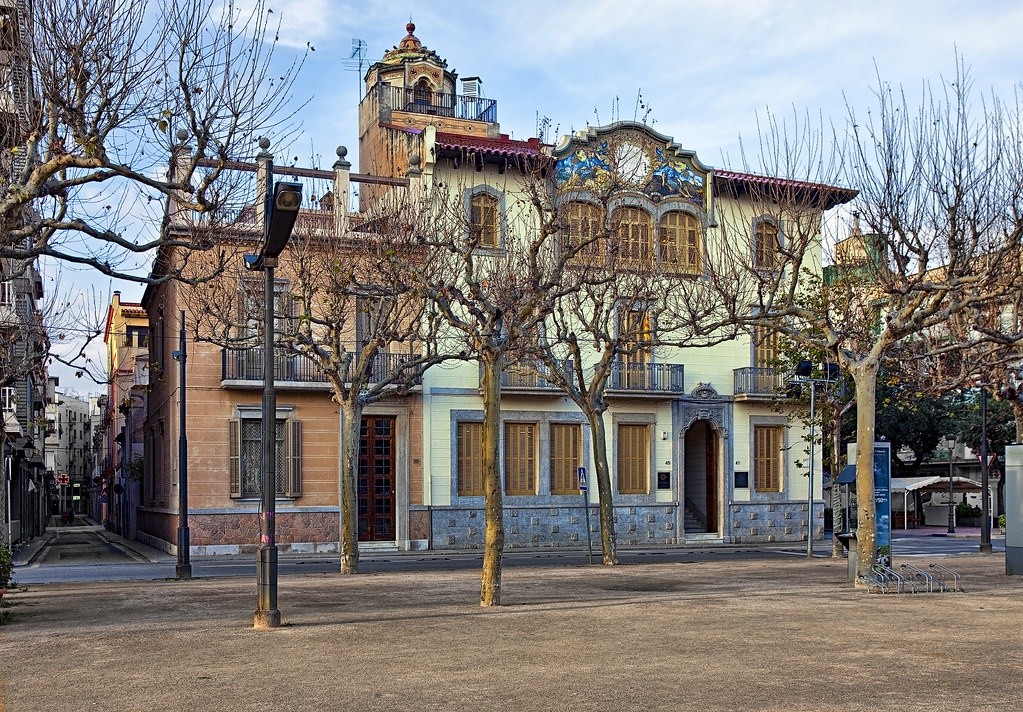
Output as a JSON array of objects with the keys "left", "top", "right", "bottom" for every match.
[{"left": 823, "top": 477, "right": 993, "bottom": 530}]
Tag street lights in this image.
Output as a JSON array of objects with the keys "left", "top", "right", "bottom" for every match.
[
  {"left": 790, "top": 361, "right": 839, "bottom": 559},
  {"left": 244, "top": 159, "right": 303, "bottom": 627},
  {"left": 946, "top": 434, "right": 959, "bottom": 534},
  {"left": 172, "top": 310, "right": 192, "bottom": 580}
]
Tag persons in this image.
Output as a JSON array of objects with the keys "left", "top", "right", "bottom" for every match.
[
  {"left": 61, "top": 511, "right": 75, "bottom": 526},
  {"left": 55, "top": 519, "right": 59, "bottom": 527}
]
